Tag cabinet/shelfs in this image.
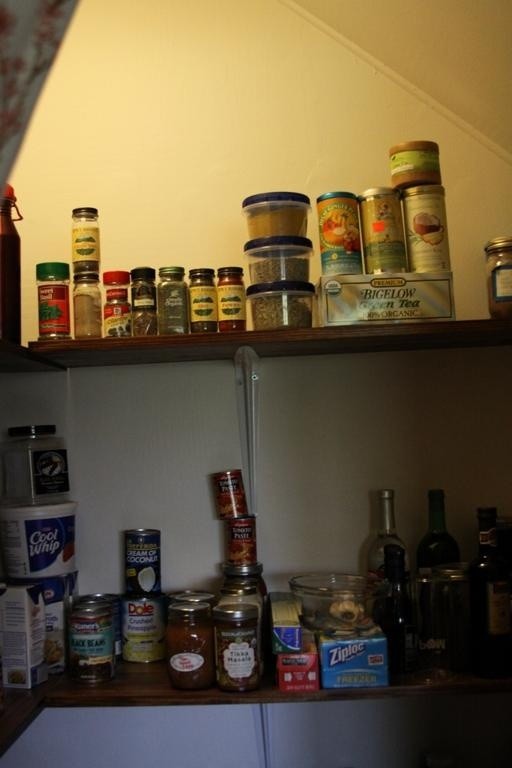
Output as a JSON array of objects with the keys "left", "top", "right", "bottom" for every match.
[{"left": 0, "top": 315, "right": 510, "bottom": 753}]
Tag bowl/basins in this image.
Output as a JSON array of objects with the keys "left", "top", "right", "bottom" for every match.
[
  {"left": 239, "top": 191, "right": 317, "bottom": 333},
  {"left": 288, "top": 569, "right": 379, "bottom": 633}
]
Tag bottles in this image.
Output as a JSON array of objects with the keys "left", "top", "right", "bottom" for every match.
[
  {"left": 167, "top": 560, "right": 270, "bottom": 694},
  {"left": 370, "top": 483, "right": 509, "bottom": 678},
  {"left": 2, "top": 425, "right": 72, "bottom": 504},
  {"left": 33, "top": 206, "right": 247, "bottom": 342}
]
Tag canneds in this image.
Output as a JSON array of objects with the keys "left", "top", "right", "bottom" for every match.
[
  {"left": 208, "top": 469, "right": 247, "bottom": 520},
  {"left": 167, "top": 590, "right": 216, "bottom": 611},
  {"left": 213, "top": 603, "right": 258, "bottom": 693},
  {"left": 167, "top": 602, "right": 214, "bottom": 691},
  {"left": 217, "top": 586, "right": 263, "bottom": 676},
  {"left": 67, "top": 593, "right": 119, "bottom": 685},
  {"left": 124, "top": 528, "right": 161, "bottom": 596},
  {"left": 224, "top": 515, "right": 256, "bottom": 565},
  {"left": 120, "top": 593, "right": 167, "bottom": 664},
  {"left": 222, "top": 562, "right": 269, "bottom": 654}
]
